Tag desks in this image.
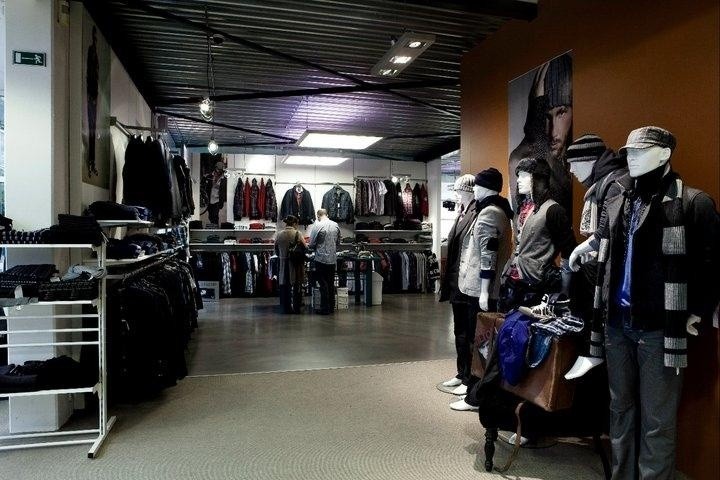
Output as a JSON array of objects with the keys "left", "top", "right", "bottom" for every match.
[{"left": 470, "top": 310, "right": 610, "bottom": 480}]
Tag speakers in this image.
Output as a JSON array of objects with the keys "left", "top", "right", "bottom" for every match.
[{"left": 158, "top": 116, "right": 168, "bottom": 129}]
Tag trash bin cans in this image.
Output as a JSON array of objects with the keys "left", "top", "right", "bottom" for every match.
[{"left": 372, "top": 272, "right": 383, "bottom": 304}]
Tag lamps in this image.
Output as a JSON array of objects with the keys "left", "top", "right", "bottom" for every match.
[
  {"left": 208, "top": 125, "right": 218, "bottom": 155},
  {"left": 199, "top": 8, "right": 216, "bottom": 122}
]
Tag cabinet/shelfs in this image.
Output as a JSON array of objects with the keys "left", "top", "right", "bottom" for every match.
[
  {"left": 0, "top": 215, "right": 204, "bottom": 460},
  {"left": 190, "top": 221, "right": 434, "bottom": 302}
]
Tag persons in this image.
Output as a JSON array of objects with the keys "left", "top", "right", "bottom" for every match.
[
  {"left": 274, "top": 215, "right": 308, "bottom": 315},
  {"left": 310, "top": 209, "right": 341, "bottom": 316},
  {"left": 443, "top": 173, "right": 479, "bottom": 394},
  {"left": 508, "top": 52, "right": 573, "bottom": 245},
  {"left": 568, "top": 135, "right": 618, "bottom": 357},
  {"left": 602, "top": 125, "right": 716, "bottom": 480},
  {"left": 500, "top": 159, "right": 565, "bottom": 444},
  {"left": 205, "top": 161, "right": 227, "bottom": 230},
  {"left": 450, "top": 168, "right": 513, "bottom": 410}
]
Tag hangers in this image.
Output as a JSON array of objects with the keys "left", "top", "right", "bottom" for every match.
[
  {"left": 356, "top": 175, "right": 427, "bottom": 185},
  {"left": 237, "top": 174, "right": 272, "bottom": 182},
  {"left": 130, "top": 127, "right": 167, "bottom": 139}
]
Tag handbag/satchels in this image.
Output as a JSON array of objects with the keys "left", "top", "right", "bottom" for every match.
[
  {"left": 513, "top": 279, "right": 541, "bottom": 306},
  {"left": 288, "top": 243, "right": 303, "bottom": 256},
  {"left": 542, "top": 265, "right": 562, "bottom": 293},
  {"left": 530, "top": 315, "right": 585, "bottom": 337}
]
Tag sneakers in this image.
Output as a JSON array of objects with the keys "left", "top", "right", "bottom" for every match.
[
  {"left": 519, "top": 302, "right": 556, "bottom": 319},
  {"left": 315, "top": 310, "right": 321, "bottom": 314}
]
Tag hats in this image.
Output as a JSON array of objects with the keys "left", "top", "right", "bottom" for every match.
[
  {"left": 453, "top": 174, "right": 476, "bottom": 193},
  {"left": 474, "top": 168, "right": 502, "bottom": 192},
  {"left": 618, "top": 126, "right": 676, "bottom": 155},
  {"left": 566, "top": 134, "right": 606, "bottom": 164},
  {"left": 515, "top": 158, "right": 551, "bottom": 175},
  {"left": 545, "top": 54, "right": 572, "bottom": 110},
  {"left": 215, "top": 162, "right": 225, "bottom": 169}
]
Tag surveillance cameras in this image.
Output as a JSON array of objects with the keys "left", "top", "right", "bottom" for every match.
[{"left": 212, "top": 34, "right": 224, "bottom": 44}]
